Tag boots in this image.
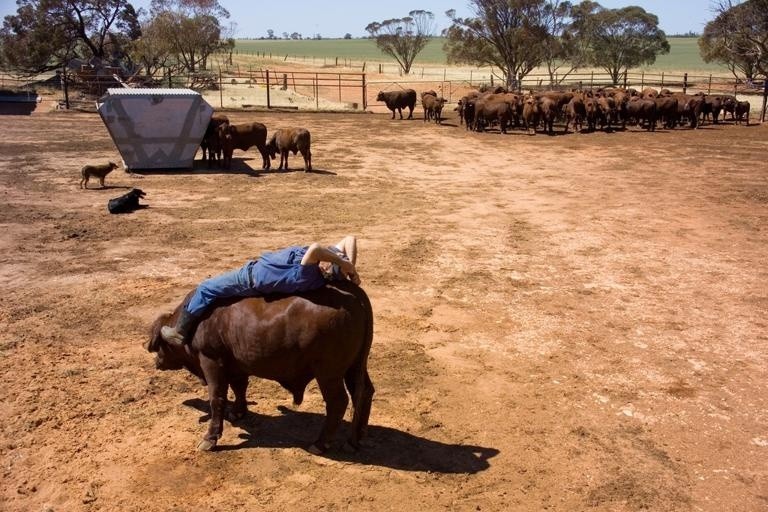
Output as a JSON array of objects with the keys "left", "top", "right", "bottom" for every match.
[{"left": 160, "top": 309, "right": 197, "bottom": 347}]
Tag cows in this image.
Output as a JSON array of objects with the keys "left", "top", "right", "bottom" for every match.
[
  {"left": 197, "top": 113, "right": 230, "bottom": 164},
  {"left": 420, "top": 88, "right": 448, "bottom": 124},
  {"left": 216, "top": 121, "right": 271, "bottom": 171},
  {"left": 453, "top": 82, "right": 750, "bottom": 136},
  {"left": 148, "top": 276, "right": 375, "bottom": 454},
  {"left": 266, "top": 126, "right": 312, "bottom": 173},
  {"left": 376, "top": 89, "right": 417, "bottom": 121}
]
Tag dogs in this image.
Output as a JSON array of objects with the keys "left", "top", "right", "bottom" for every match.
[
  {"left": 80, "top": 161, "right": 119, "bottom": 189},
  {"left": 107, "top": 188, "right": 150, "bottom": 214}
]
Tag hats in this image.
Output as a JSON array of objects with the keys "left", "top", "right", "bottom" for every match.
[{"left": 327, "top": 245, "right": 349, "bottom": 279}]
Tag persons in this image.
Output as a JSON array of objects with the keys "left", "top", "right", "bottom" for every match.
[{"left": 151, "top": 230, "right": 360, "bottom": 347}]
